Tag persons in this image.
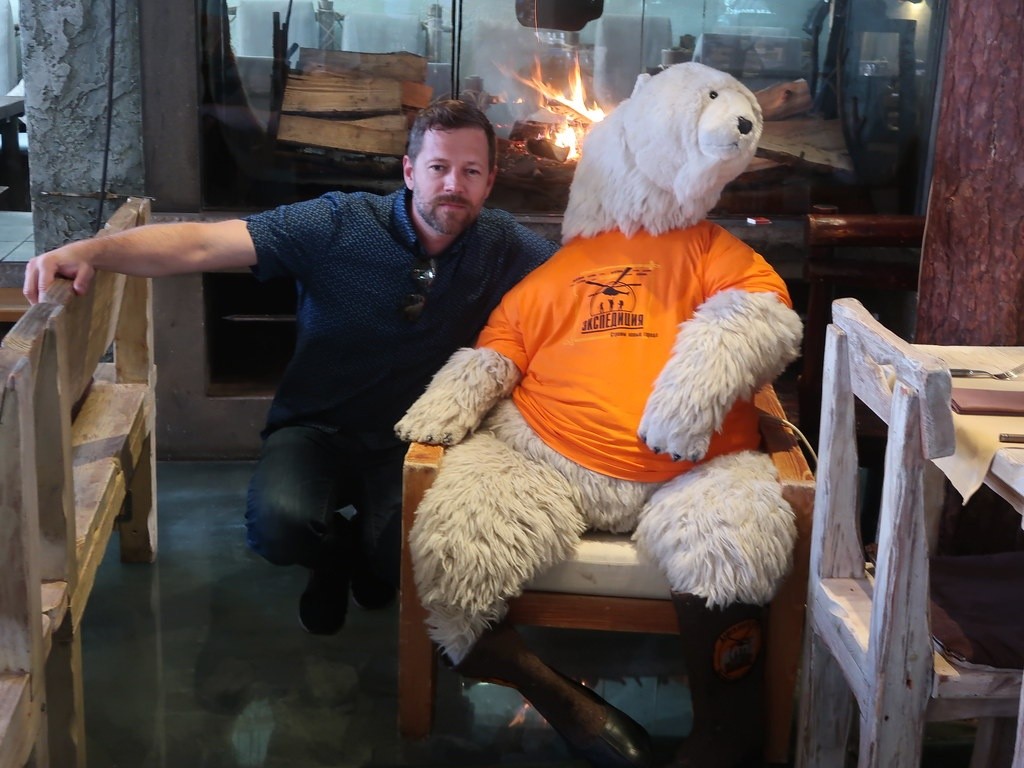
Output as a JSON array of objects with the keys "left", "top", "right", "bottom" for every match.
[{"left": 23, "top": 99, "right": 564, "bottom": 635}]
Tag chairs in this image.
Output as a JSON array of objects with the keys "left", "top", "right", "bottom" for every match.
[
  {"left": 592, "top": 14, "right": 673, "bottom": 111},
  {"left": 396, "top": 384, "right": 815, "bottom": 768},
  {"left": 793, "top": 298, "right": 1024, "bottom": 768},
  {"left": 341, "top": 12, "right": 424, "bottom": 55}
]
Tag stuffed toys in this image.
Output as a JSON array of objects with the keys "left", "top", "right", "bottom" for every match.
[{"left": 393, "top": 62, "right": 803, "bottom": 666}]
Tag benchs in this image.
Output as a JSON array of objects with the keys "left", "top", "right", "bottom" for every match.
[
  {"left": 0, "top": 346, "right": 50, "bottom": 768},
  {"left": 0, "top": 195, "right": 158, "bottom": 768}
]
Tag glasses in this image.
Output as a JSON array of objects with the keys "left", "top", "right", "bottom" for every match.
[{"left": 403, "top": 264, "right": 436, "bottom": 322}]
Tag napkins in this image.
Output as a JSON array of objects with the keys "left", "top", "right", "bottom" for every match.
[{"left": 889, "top": 373, "right": 1024, "bottom": 506}]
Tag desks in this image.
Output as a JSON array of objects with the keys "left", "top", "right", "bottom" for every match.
[{"left": 881, "top": 344, "right": 1024, "bottom": 556}]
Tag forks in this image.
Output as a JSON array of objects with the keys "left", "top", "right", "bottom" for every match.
[{"left": 949, "top": 363, "right": 1024, "bottom": 381}]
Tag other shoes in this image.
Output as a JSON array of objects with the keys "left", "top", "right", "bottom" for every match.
[
  {"left": 345, "top": 555, "right": 396, "bottom": 611},
  {"left": 299, "top": 570, "right": 351, "bottom": 634}
]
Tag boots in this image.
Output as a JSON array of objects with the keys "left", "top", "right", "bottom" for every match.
[
  {"left": 450, "top": 623, "right": 653, "bottom": 768},
  {"left": 671, "top": 592, "right": 770, "bottom": 768}
]
